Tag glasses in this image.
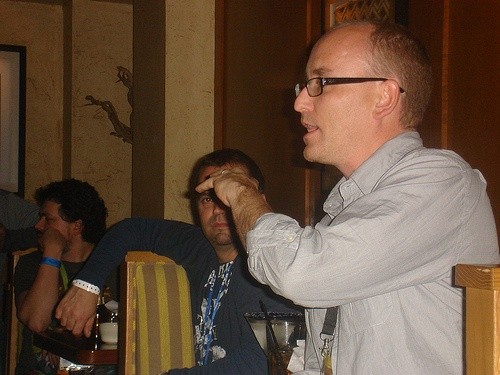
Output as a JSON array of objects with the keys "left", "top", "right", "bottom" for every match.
[{"left": 295, "top": 77, "right": 405, "bottom": 97}]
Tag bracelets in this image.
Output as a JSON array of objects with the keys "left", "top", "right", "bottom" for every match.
[
  {"left": 42, "top": 257, "right": 61, "bottom": 268},
  {"left": 71, "top": 279, "right": 100, "bottom": 295}
]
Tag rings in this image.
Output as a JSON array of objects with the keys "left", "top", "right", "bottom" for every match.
[{"left": 221, "top": 169, "right": 226, "bottom": 174}]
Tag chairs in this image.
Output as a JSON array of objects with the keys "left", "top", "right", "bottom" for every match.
[{"left": 118, "top": 251, "right": 196, "bottom": 375}]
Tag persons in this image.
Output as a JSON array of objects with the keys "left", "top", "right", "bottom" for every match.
[
  {"left": 56, "top": 150, "right": 305, "bottom": 375},
  {"left": 13, "top": 178, "right": 108, "bottom": 375},
  {"left": 0, "top": 188, "right": 42, "bottom": 375},
  {"left": 196, "top": 19, "right": 499, "bottom": 375}
]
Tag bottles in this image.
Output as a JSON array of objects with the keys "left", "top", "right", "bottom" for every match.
[{"left": 91, "top": 293, "right": 104, "bottom": 340}]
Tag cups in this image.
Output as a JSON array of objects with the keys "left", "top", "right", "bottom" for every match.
[{"left": 100, "top": 322, "right": 118, "bottom": 344}]
